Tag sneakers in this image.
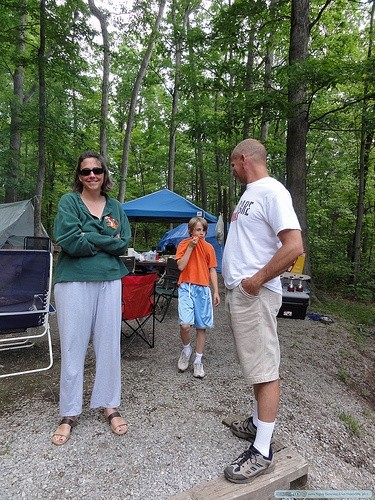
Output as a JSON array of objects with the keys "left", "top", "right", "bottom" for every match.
[
  {"left": 192, "top": 361, "right": 204, "bottom": 377},
  {"left": 231, "top": 417, "right": 273, "bottom": 445},
  {"left": 177, "top": 347, "right": 193, "bottom": 371},
  {"left": 224, "top": 443, "right": 275, "bottom": 484}
]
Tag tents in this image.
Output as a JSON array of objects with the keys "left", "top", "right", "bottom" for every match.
[
  {"left": 120, "top": 189, "right": 218, "bottom": 224},
  {"left": 156, "top": 223, "right": 231, "bottom": 273},
  {"left": 0, "top": 199, "right": 54, "bottom": 250}
]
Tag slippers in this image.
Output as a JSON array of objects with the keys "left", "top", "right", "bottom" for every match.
[
  {"left": 103, "top": 408, "right": 128, "bottom": 435},
  {"left": 51, "top": 416, "right": 77, "bottom": 445}
]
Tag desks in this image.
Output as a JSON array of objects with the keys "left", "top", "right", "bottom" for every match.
[{"left": 134, "top": 262, "right": 166, "bottom": 274}]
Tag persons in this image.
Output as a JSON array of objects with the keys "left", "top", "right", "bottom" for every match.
[
  {"left": 51, "top": 152, "right": 132, "bottom": 446},
  {"left": 221, "top": 138, "right": 304, "bottom": 484},
  {"left": 175, "top": 217, "right": 221, "bottom": 379}
]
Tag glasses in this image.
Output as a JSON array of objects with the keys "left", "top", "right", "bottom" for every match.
[{"left": 77, "top": 168, "right": 106, "bottom": 176}]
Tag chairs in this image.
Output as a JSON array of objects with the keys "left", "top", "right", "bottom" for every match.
[{"left": 0, "top": 236, "right": 181, "bottom": 379}]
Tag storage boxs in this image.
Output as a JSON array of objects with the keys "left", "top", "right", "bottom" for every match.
[{"left": 277, "top": 290, "right": 311, "bottom": 320}]
